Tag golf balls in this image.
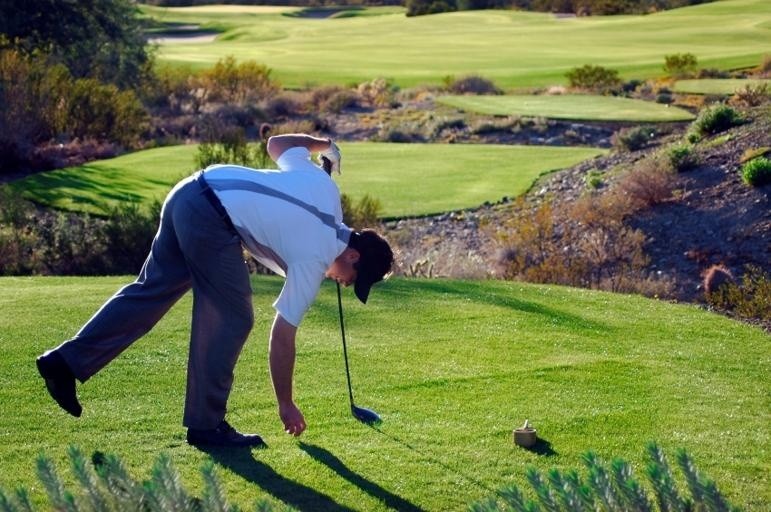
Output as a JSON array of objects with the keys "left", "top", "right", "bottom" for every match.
[{"left": 289, "top": 423, "right": 304, "bottom": 433}]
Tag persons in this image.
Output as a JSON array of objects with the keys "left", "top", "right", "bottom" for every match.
[{"left": 33, "top": 132, "right": 394, "bottom": 451}]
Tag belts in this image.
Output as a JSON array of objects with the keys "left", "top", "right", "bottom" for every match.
[{"left": 192, "top": 168, "right": 238, "bottom": 238}]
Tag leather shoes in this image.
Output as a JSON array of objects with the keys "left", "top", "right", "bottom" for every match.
[
  {"left": 35, "top": 351, "right": 83, "bottom": 418},
  {"left": 185, "top": 417, "right": 263, "bottom": 449}
]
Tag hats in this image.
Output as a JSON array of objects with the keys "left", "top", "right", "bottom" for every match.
[{"left": 353, "top": 223, "right": 394, "bottom": 305}]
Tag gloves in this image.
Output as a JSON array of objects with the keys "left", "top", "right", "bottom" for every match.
[{"left": 316, "top": 136, "right": 345, "bottom": 178}]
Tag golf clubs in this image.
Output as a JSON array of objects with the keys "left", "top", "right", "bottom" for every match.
[{"left": 320, "top": 156, "right": 383, "bottom": 424}]
